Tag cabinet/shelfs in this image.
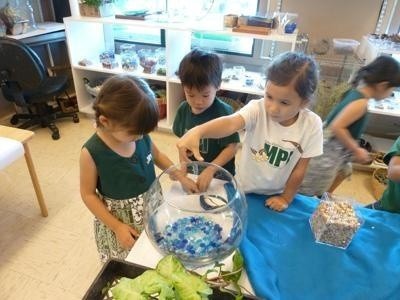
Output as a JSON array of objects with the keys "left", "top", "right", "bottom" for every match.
[{"left": 62, "top": 15, "right": 299, "bottom": 138}]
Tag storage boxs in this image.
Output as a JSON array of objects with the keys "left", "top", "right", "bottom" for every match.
[{"left": 333, "top": 33, "right": 399, "bottom": 141}]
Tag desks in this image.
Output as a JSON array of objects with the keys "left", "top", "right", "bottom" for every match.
[
  {"left": 123, "top": 169, "right": 400, "bottom": 300},
  {"left": 0, "top": 124, "right": 49, "bottom": 217},
  {"left": 0, "top": 20, "right": 77, "bottom": 106}
]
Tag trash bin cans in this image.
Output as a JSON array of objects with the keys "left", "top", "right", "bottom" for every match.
[{"left": 153, "top": 89, "right": 166, "bottom": 119}]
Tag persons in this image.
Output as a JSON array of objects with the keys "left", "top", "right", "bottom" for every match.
[
  {"left": 176, "top": 51, "right": 323, "bottom": 212},
  {"left": 380, "top": 136, "right": 400, "bottom": 212},
  {"left": 297, "top": 55, "right": 400, "bottom": 199},
  {"left": 79, "top": 74, "right": 200, "bottom": 264},
  {"left": 172, "top": 48, "right": 240, "bottom": 193}
]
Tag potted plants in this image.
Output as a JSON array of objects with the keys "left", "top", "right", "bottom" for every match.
[
  {"left": 81, "top": 247, "right": 261, "bottom": 300},
  {"left": 1, "top": 1, "right": 30, "bottom": 35}
]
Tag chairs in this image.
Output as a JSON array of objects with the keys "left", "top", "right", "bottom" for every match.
[{"left": 1, "top": 36, "right": 80, "bottom": 139}]
